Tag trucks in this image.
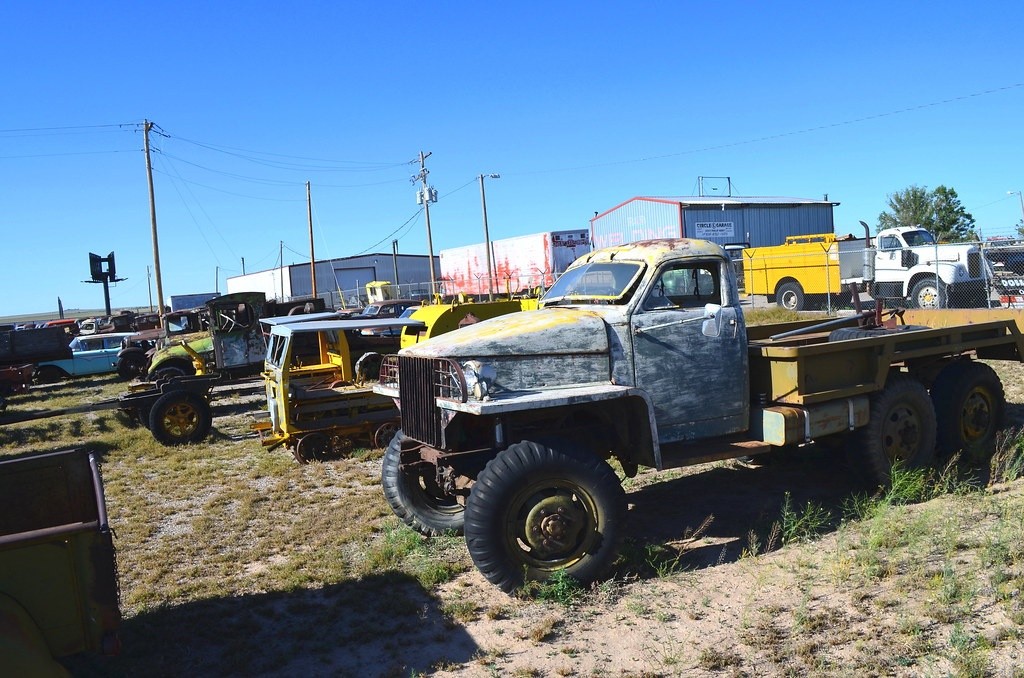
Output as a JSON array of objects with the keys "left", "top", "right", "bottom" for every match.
[
  {"left": 742, "top": 221, "right": 993, "bottom": 312},
  {"left": 380, "top": 237, "right": 1024, "bottom": 601}
]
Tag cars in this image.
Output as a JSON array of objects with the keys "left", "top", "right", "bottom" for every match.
[{"left": 31, "top": 332, "right": 137, "bottom": 385}]
